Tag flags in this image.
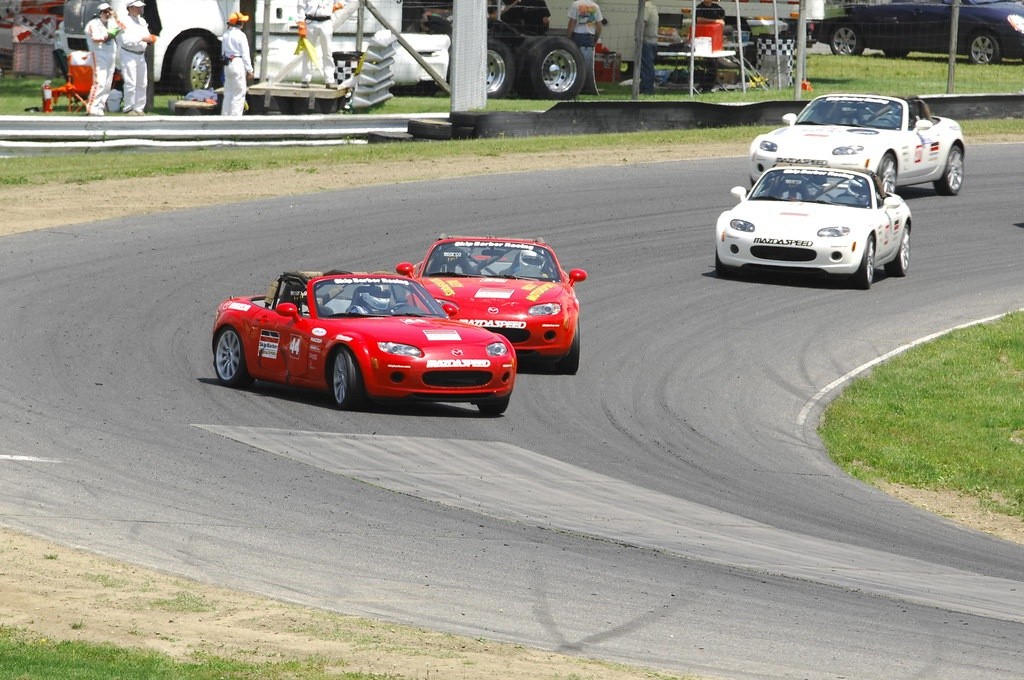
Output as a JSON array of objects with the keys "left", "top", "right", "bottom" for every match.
[{"left": 294, "top": 37, "right": 317, "bottom": 65}]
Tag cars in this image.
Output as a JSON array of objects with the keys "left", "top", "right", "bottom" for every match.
[
  {"left": 749, "top": 93, "right": 968, "bottom": 199},
  {"left": 716, "top": 162, "right": 913, "bottom": 288},
  {"left": 396, "top": 232, "right": 588, "bottom": 376},
  {"left": 812, "top": 1, "right": 1024, "bottom": 66},
  {"left": 209, "top": 268, "right": 516, "bottom": 416}
]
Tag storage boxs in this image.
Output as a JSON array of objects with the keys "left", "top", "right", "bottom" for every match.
[{"left": 751, "top": 24, "right": 787, "bottom": 36}]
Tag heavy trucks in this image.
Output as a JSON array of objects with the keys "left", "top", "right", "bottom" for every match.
[{"left": 54, "top": 0, "right": 602, "bottom": 100}]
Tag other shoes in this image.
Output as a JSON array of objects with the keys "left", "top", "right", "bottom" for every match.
[
  {"left": 326, "top": 83, "right": 338, "bottom": 90},
  {"left": 302, "top": 81, "right": 309, "bottom": 88}
]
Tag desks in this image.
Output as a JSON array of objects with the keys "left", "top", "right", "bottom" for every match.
[
  {"left": 657, "top": 51, "right": 737, "bottom": 91},
  {"left": 646, "top": 39, "right": 768, "bottom": 88}
]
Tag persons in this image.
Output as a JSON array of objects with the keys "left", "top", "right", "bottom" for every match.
[
  {"left": 297, "top": 0, "right": 346, "bottom": 89},
  {"left": 511, "top": 250, "right": 548, "bottom": 278},
  {"left": 347, "top": 284, "right": 397, "bottom": 316},
  {"left": 636, "top": 0, "right": 659, "bottom": 94},
  {"left": 488, "top": 0, "right": 551, "bottom": 28},
  {"left": 567, "top": 0, "right": 604, "bottom": 48},
  {"left": 221, "top": 11, "right": 255, "bottom": 115},
  {"left": 84, "top": 0, "right": 158, "bottom": 116},
  {"left": 692, "top": 0, "right": 726, "bottom": 91},
  {"left": 54, "top": 21, "right": 66, "bottom": 78},
  {"left": 836, "top": 177, "right": 878, "bottom": 209},
  {"left": 887, "top": 103, "right": 917, "bottom": 128}
]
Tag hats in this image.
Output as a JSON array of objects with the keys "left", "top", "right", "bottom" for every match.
[
  {"left": 228, "top": 12, "right": 249, "bottom": 25},
  {"left": 126, "top": 0, "right": 146, "bottom": 7},
  {"left": 97, "top": 3, "right": 110, "bottom": 10}
]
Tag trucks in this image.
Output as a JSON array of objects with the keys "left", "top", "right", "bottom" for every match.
[{"left": 493, "top": 0, "right": 849, "bottom": 68}]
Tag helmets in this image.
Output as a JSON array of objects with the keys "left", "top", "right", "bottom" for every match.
[
  {"left": 362, "top": 284, "right": 392, "bottom": 310},
  {"left": 519, "top": 250, "right": 545, "bottom": 270},
  {"left": 847, "top": 180, "right": 862, "bottom": 197}
]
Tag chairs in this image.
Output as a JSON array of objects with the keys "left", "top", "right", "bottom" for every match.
[
  {"left": 832, "top": 171, "right": 878, "bottom": 208},
  {"left": 771, "top": 174, "right": 811, "bottom": 201},
  {"left": 828, "top": 102, "right": 864, "bottom": 124},
  {"left": 500, "top": 251, "right": 547, "bottom": 279},
  {"left": 42, "top": 49, "right": 99, "bottom": 113},
  {"left": 901, "top": 99, "right": 931, "bottom": 125},
  {"left": 428, "top": 247, "right": 474, "bottom": 278},
  {"left": 347, "top": 286, "right": 384, "bottom": 314}
]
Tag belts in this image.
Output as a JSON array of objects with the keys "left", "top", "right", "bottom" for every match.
[
  {"left": 121, "top": 47, "right": 146, "bottom": 55},
  {"left": 305, "top": 14, "right": 331, "bottom": 22}
]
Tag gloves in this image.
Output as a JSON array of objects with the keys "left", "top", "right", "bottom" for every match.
[
  {"left": 297, "top": 21, "right": 307, "bottom": 38},
  {"left": 332, "top": 2, "right": 343, "bottom": 12}
]
